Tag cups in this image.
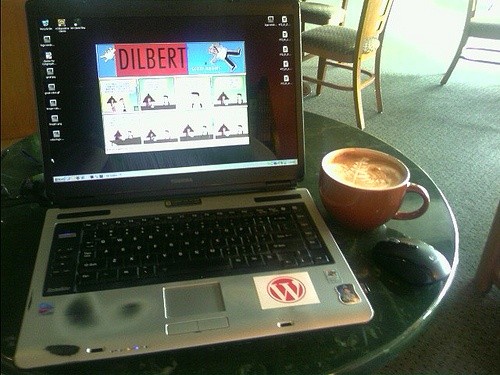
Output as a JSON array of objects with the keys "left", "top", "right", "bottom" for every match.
[{"left": 320, "top": 147, "right": 429, "bottom": 230}]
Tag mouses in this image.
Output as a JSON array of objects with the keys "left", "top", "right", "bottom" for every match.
[{"left": 375, "top": 237, "right": 452, "bottom": 285}]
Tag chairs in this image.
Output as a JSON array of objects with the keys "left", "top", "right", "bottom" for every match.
[
  {"left": 300, "top": 0, "right": 394, "bottom": 131},
  {"left": 440, "top": 0, "right": 500, "bottom": 87}
]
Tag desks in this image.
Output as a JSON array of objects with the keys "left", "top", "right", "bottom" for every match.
[{"left": 0, "top": 110, "right": 459, "bottom": 375}]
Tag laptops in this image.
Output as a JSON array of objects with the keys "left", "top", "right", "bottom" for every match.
[{"left": 14, "top": 0, "right": 374, "bottom": 370}]
